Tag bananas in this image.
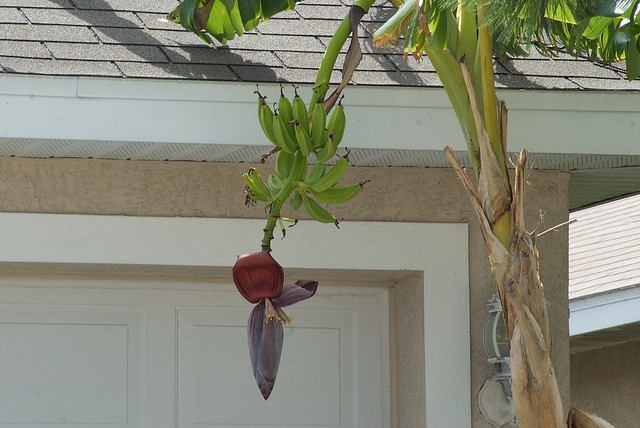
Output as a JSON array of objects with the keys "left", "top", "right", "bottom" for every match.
[
  {"left": 268, "top": 150, "right": 371, "bottom": 229},
  {"left": 252, "top": 83, "right": 347, "bottom": 162},
  {"left": 240, "top": 166, "right": 276, "bottom": 204}
]
